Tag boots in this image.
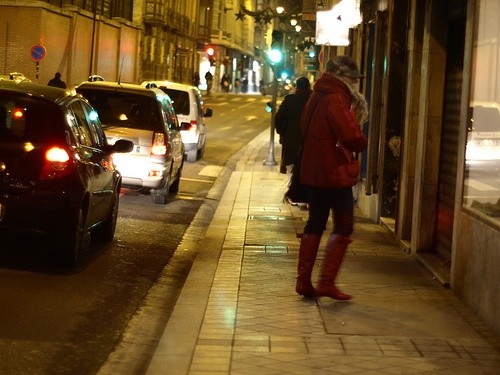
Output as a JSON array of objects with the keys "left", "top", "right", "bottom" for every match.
[
  {"left": 295, "top": 232, "right": 323, "bottom": 297},
  {"left": 316, "top": 232, "right": 353, "bottom": 300}
]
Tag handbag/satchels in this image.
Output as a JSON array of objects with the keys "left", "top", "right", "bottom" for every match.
[{"left": 282, "top": 153, "right": 309, "bottom": 206}]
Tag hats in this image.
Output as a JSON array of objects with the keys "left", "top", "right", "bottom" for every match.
[
  {"left": 295, "top": 76, "right": 311, "bottom": 89},
  {"left": 326, "top": 55, "right": 364, "bottom": 79}
]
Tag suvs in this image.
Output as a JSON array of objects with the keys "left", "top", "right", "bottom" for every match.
[
  {"left": 464, "top": 100, "right": 500, "bottom": 171},
  {"left": 71, "top": 74, "right": 192, "bottom": 204},
  {"left": 0, "top": 71, "right": 134, "bottom": 271}
]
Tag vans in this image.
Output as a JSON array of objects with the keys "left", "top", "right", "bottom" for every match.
[{"left": 139, "top": 80, "right": 213, "bottom": 163}]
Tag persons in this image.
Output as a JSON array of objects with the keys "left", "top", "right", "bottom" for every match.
[
  {"left": 274, "top": 77, "right": 314, "bottom": 210},
  {"left": 221, "top": 71, "right": 265, "bottom": 95},
  {"left": 205, "top": 71, "right": 213, "bottom": 94},
  {"left": 295, "top": 55, "right": 369, "bottom": 300},
  {"left": 192, "top": 72, "right": 200, "bottom": 87},
  {"left": 48, "top": 72, "right": 66, "bottom": 89}
]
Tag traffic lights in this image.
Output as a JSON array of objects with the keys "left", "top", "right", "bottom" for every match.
[
  {"left": 267, "top": 29, "right": 282, "bottom": 64},
  {"left": 207, "top": 47, "right": 215, "bottom": 63}
]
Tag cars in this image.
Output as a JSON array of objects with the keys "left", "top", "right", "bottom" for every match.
[{"left": 260, "top": 79, "right": 288, "bottom": 98}]
